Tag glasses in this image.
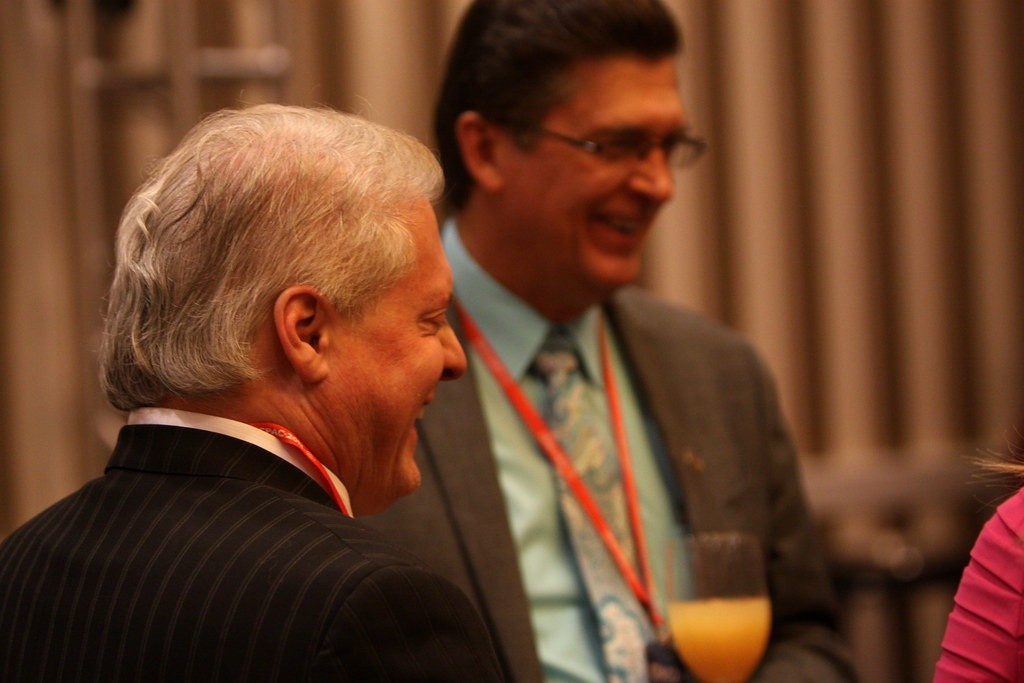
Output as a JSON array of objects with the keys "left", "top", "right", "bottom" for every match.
[{"left": 500, "top": 118, "right": 704, "bottom": 170}]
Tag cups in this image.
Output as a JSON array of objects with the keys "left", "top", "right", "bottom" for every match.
[{"left": 663, "top": 532, "right": 772, "bottom": 683}]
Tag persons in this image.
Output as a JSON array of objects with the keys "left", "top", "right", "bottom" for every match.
[
  {"left": 353, "top": 0, "right": 863, "bottom": 683},
  {"left": 931, "top": 431, "right": 1024, "bottom": 683},
  {"left": 1, "top": 101, "right": 521, "bottom": 682}
]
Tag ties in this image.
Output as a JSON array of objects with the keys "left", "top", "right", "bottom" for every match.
[{"left": 529, "top": 331, "right": 655, "bottom": 682}]
